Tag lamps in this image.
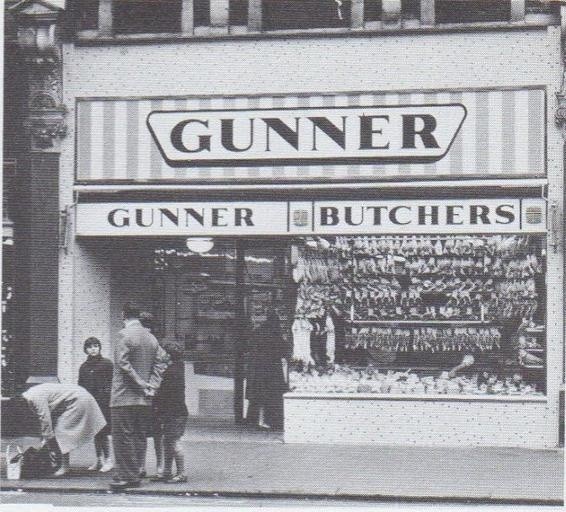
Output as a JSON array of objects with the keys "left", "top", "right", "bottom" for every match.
[{"left": 185, "top": 237, "right": 214, "bottom": 253}]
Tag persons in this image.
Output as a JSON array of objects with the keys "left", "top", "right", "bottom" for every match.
[
  {"left": 77, "top": 303, "right": 190, "bottom": 490},
  {"left": 242, "top": 308, "right": 292, "bottom": 435},
  {"left": 7, "top": 382, "right": 106, "bottom": 478}
]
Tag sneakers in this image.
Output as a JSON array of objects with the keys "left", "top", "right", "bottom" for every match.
[{"left": 43, "top": 455, "right": 193, "bottom": 491}]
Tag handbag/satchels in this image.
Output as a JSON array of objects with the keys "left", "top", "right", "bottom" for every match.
[
  {"left": 5, "top": 441, "right": 22, "bottom": 479},
  {"left": 23, "top": 445, "right": 38, "bottom": 476}
]
